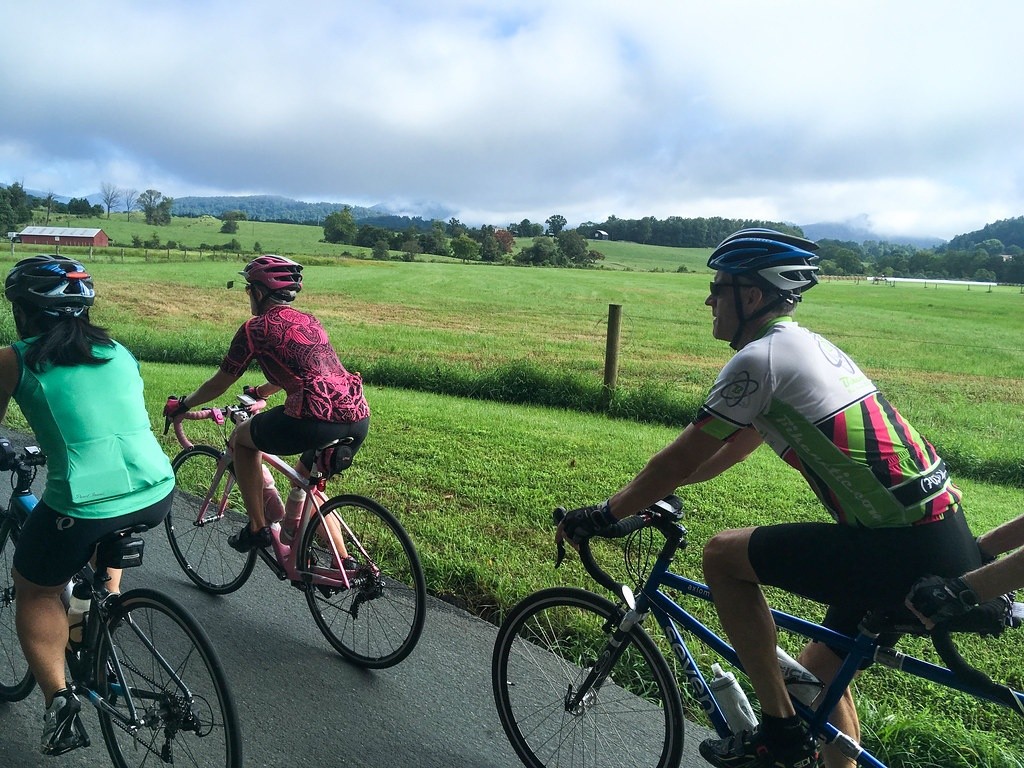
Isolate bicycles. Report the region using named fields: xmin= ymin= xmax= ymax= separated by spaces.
xmin=162 ymin=385 xmax=429 ymax=670
xmin=491 ymin=493 xmax=1024 ymax=768
xmin=0 ymin=436 xmax=243 ymax=768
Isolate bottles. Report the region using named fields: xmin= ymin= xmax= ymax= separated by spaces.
xmin=777 ymin=646 xmax=825 ymax=705
xmin=67 ymin=579 xmax=91 ymax=642
xmin=280 ymin=485 xmax=307 ymax=545
xmin=709 ymin=662 xmax=759 ymax=735
xmin=261 ymin=464 xmax=285 ymax=522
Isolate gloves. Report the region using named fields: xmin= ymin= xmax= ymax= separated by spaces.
xmin=243 ymin=385 xmax=269 ymax=402
xmin=163 ymin=396 xmax=190 ymax=417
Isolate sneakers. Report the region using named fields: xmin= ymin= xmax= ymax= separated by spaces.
xmin=41 ymin=688 xmax=81 ymax=755
xmin=330 ymin=555 xmax=357 ymax=571
xmin=699 ymin=724 xmax=817 ymax=768
xmin=227 ymin=522 xmax=273 ymax=552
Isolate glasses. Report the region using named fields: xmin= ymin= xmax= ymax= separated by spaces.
xmin=710 ymin=281 xmax=766 ymax=298
xmin=245 ymin=286 xmax=264 ymax=296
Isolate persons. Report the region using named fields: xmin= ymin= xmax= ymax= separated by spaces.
xmin=161 ymin=253 xmax=373 ymax=571
xmin=554 ymin=226 xmax=982 ymax=768
xmin=0 ymin=253 xmax=175 ymax=755
xmin=907 ymin=513 xmax=1024 ymax=630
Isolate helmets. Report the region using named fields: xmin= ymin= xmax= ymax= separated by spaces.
xmin=238 ymin=255 xmax=303 ymax=292
xmin=5 ymin=254 xmax=95 ymax=317
xmin=706 ymin=227 xmax=820 ymax=305
